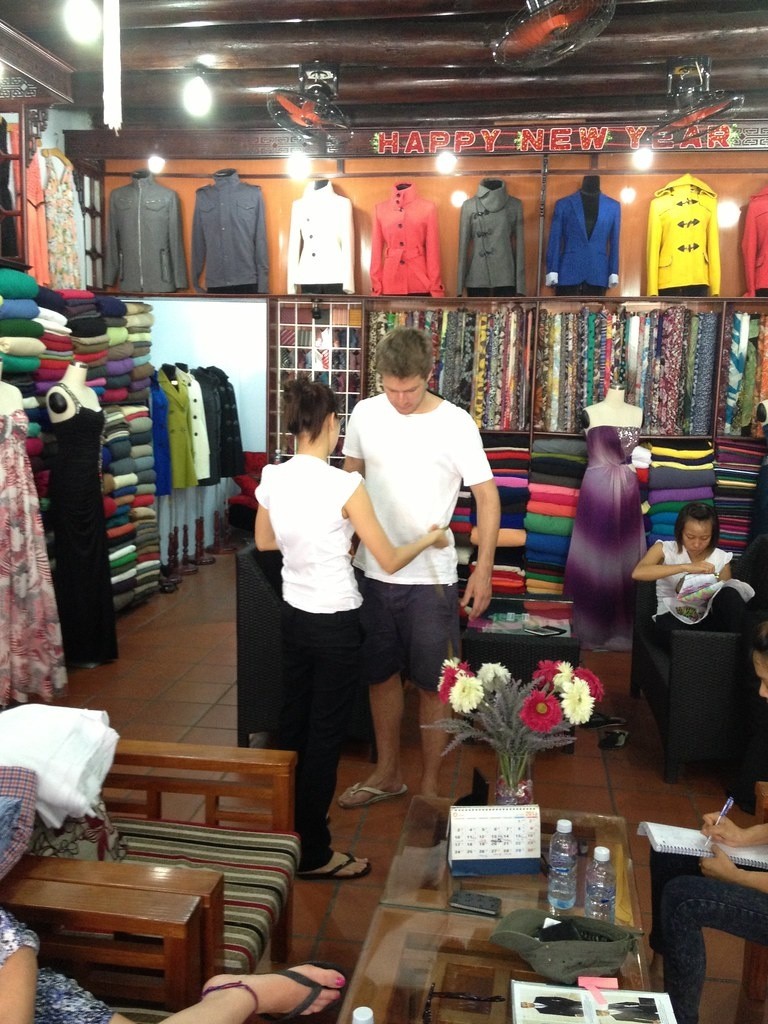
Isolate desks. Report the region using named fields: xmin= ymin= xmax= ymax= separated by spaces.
xmin=454 ymin=597 xmax=582 ymax=755
xmin=378 ymin=795 xmax=644 ymax=935
xmin=337 ymin=903 xmax=652 ymax=1024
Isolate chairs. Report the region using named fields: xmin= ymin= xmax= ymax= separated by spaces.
xmin=0 ymin=702 xmax=300 ymax=1024
xmin=234 ymin=542 xmax=408 ymax=764
xmin=628 ymin=534 xmax=768 ymax=783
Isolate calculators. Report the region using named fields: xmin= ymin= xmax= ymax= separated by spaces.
xmin=525 ymin=626 xmax=566 ymax=636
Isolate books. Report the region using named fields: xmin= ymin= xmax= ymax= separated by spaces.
xmin=637 ymin=821 xmax=768 ymax=869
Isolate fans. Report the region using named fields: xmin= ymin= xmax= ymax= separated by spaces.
xmin=490 ymin=0 xmax=617 ymax=72
xmin=645 ymin=56 xmax=744 ymax=145
xmin=263 ymin=61 xmax=354 ymax=149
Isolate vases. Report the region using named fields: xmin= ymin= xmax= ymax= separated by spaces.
xmin=493 ymin=774 xmax=534 ymax=806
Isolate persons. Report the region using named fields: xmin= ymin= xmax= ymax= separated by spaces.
xmin=40 ymin=358 xmax=120 ymax=668
xmin=0 ymin=905 xmax=346 ymax=1024
xmin=546 ymin=173 xmax=621 ymax=297
xmin=595 ymin=1001 xmax=662 ymax=1024
xmin=106 ymin=169 xmax=189 ymax=294
xmin=521 ymin=995 xmax=583 ymax=1018
xmin=566 ymin=382 xmax=648 ymax=652
xmin=190 ymin=364 xmax=246 ymax=554
xmin=370 ymin=182 xmax=446 ymax=298
xmin=148 ymin=360 xmax=212 ymax=571
xmin=648 ymin=624 xmax=768 ymax=1024
xmin=741 ymin=186 xmax=768 ymax=296
xmin=0 ymin=359 xmax=68 ymax=715
xmin=454 ymin=178 xmax=527 ymax=298
xmin=631 ymin=502 xmax=733 ymax=650
xmin=337 ymin=327 xmax=500 ymax=813
xmin=286 ymin=179 xmax=355 ymax=296
xmin=254 ymin=380 xmax=449 ymax=879
xmin=189 ymin=168 xmax=269 ymax=294
xmin=647 ymin=172 xmax=722 ymax=295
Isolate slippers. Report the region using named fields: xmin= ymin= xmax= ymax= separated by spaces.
xmin=203 ymin=961 xmax=346 ymax=1022
xmin=579 ymin=711 xmax=627 ymax=730
xmin=338 ymin=781 xmax=409 ymax=808
xmin=296 ymin=851 xmax=371 ymax=877
xmin=597 ymin=729 xmax=630 ymax=748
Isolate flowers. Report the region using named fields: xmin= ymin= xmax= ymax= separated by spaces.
xmin=420 ymin=656 xmax=606 ymax=787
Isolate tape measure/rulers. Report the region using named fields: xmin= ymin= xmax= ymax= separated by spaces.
xmin=426 ymin=525 xmax=457 ymax=660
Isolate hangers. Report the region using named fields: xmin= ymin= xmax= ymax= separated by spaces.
xmin=40 ymin=132 xmax=75 ymax=172
xmin=6 ymin=123 xmax=43 ymax=147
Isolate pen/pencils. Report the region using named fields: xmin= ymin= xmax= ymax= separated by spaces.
xmin=704 ymin=796 xmax=735 ymax=845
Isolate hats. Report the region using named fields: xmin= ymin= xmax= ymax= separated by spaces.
xmin=487 ymin=907 xmax=636 ymax=983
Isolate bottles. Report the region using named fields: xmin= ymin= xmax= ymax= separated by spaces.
xmin=584 ymin=846 xmax=615 ymax=924
xmin=547 ymin=819 xmax=577 ymax=910
xmin=351 ymin=1006 xmax=374 ymax=1024
